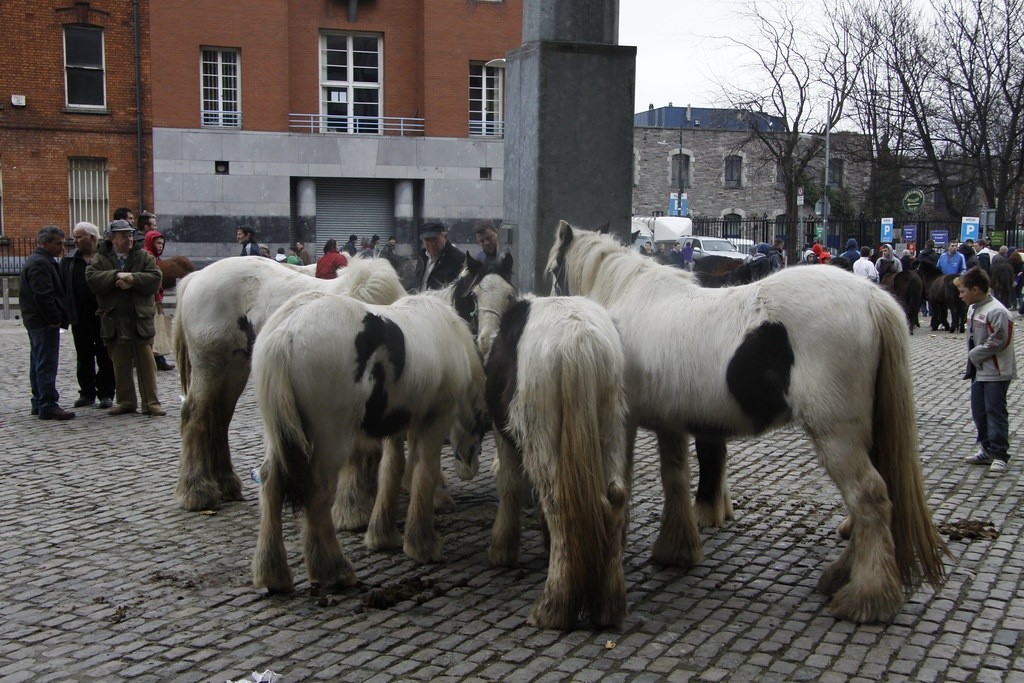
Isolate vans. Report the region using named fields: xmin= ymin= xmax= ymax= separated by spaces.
xmin=632 ymin=221 xmax=657 ymax=255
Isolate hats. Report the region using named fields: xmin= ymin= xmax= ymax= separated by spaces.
xmin=372 ymin=234 xmax=379 ymax=241
xmin=419 ymin=221 xmax=445 ymax=239
xmin=107 ymin=219 xmax=135 ymax=233
xmin=999 ymin=246 xmax=1009 ymax=252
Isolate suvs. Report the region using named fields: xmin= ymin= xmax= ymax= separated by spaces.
xmin=675 ymin=234 xmax=746 ymax=261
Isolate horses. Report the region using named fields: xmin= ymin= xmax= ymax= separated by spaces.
xmin=990 ymin=261 xmax=1014 ymax=310
xmin=156 ymin=254 xmax=409 ymax=511
xmin=254 ymin=280 xmax=486 ymax=606
xmin=879 ymin=259 xmax=922 ymax=336
xmin=693 ymin=256 xmax=773 ymax=289
xmin=456 ymin=254 xmax=631 ymax=632
xmin=911 ymin=259 xmax=969 ymax=333
xmin=546 ymin=216 xmax=954 ymax=627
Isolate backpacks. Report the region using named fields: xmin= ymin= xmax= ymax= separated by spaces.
xmin=340 ymin=242 xmax=350 ymax=253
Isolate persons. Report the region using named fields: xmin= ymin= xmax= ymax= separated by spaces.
xmin=341 ymin=235 xmax=396 ymax=260
xmin=315 ymin=239 xmax=348 ymax=280
xmin=743 ymin=238 xmax=784 ymax=283
xmin=237 ymin=224 xmax=261 ymax=256
xmin=86 ymin=220 xmax=167 ymax=416
xmin=19 ymin=226 xmax=75 ymax=419
xmin=103 ymin=208 xmax=135 ymax=241
xmin=955 ymin=266 xmax=1018 ymax=471
xmin=474 ymin=220 xmax=498 ymax=265
xmin=143 ymin=232 xmax=175 ymax=372
xmin=61 ymin=222 xmax=116 ymax=408
xmin=798 ymin=238 xmax=1024 ymax=330
xmin=134 ymin=212 xmax=157 ymax=249
xmin=644 ymin=241 xmax=693 ymax=270
xmin=258 ymin=241 xmax=312 ymax=266
xmin=414 ymin=220 xmax=467 ymax=293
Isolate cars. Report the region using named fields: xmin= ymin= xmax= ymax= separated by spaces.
xmin=724 ymin=237 xmax=760 ymax=252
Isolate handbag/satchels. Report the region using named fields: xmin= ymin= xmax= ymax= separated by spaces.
xmin=152 ymin=304 xmax=174 ymax=356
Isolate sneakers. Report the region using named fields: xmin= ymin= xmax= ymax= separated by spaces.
xmin=966 ymin=449 xmax=994 ymax=464
xmin=991 ymin=458 xmax=1008 ymax=471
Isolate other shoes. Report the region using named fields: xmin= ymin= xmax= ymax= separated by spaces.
xmin=158 ymin=363 xmax=176 ymax=371
xmin=99 ymin=397 xmax=113 ymax=408
xmin=108 ymin=405 xmax=136 ymax=415
xmin=74 ymin=395 xmax=96 ymax=406
xmin=31 ymin=404 xmax=39 ymax=414
xmin=39 ymin=407 xmax=75 ymax=420
xmin=145 ymin=409 xmax=167 ymax=416
